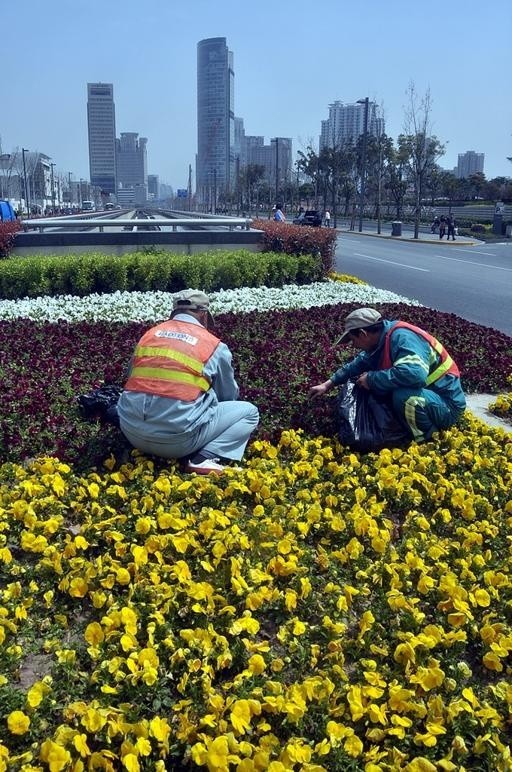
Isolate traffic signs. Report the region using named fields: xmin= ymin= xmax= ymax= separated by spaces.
xmin=177 ymin=189 xmax=188 ymax=198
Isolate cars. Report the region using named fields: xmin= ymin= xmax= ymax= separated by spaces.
xmin=116 ymin=205 xmax=121 ymax=209
xmin=96 ymin=207 xmax=105 ymax=212
xmin=209 ymin=207 xmax=229 ymax=213
xmin=292 ymin=209 xmax=323 ymax=227
xmin=42 ymin=205 xmax=80 ymax=215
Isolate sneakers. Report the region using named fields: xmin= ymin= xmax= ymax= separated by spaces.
xmin=183 ymin=457 xmax=245 ymax=477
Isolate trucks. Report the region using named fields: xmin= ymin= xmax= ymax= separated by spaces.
xmin=0 ymin=199 xmax=17 ymax=222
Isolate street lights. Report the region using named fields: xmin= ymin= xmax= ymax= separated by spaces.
xmin=68 ymin=170 xmax=73 ymax=186
xmin=22 ymin=147 xmax=29 ymax=218
xmin=50 ymin=162 xmax=56 ymax=206
xmin=271 ymin=136 xmax=280 ymax=206
xmin=79 ymin=177 xmax=83 ymax=201
xmin=356 ymin=96 xmax=373 ymax=233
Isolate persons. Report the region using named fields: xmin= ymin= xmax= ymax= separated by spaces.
xmin=306 ymin=308 xmax=466 ymax=449
xmin=273 ymin=202 xmax=330 ymax=227
xmin=430 ymin=213 xmax=456 ymax=240
xmin=116 ymin=291 xmax=260 ymax=476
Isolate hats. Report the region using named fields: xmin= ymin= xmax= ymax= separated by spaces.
xmin=170 ymin=289 xmax=216 ymax=331
xmin=335 ymin=307 xmax=384 ymax=346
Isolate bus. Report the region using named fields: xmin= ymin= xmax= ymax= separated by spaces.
xmin=81 ymin=200 xmax=96 ymax=213
xmin=105 ymin=202 xmax=114 ymax=211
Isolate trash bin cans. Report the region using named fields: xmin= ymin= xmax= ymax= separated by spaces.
xmin=242 ymin=211 xmax=247 ymax=218
xmin=391 ymin=221 xmax=402 ymax=236
